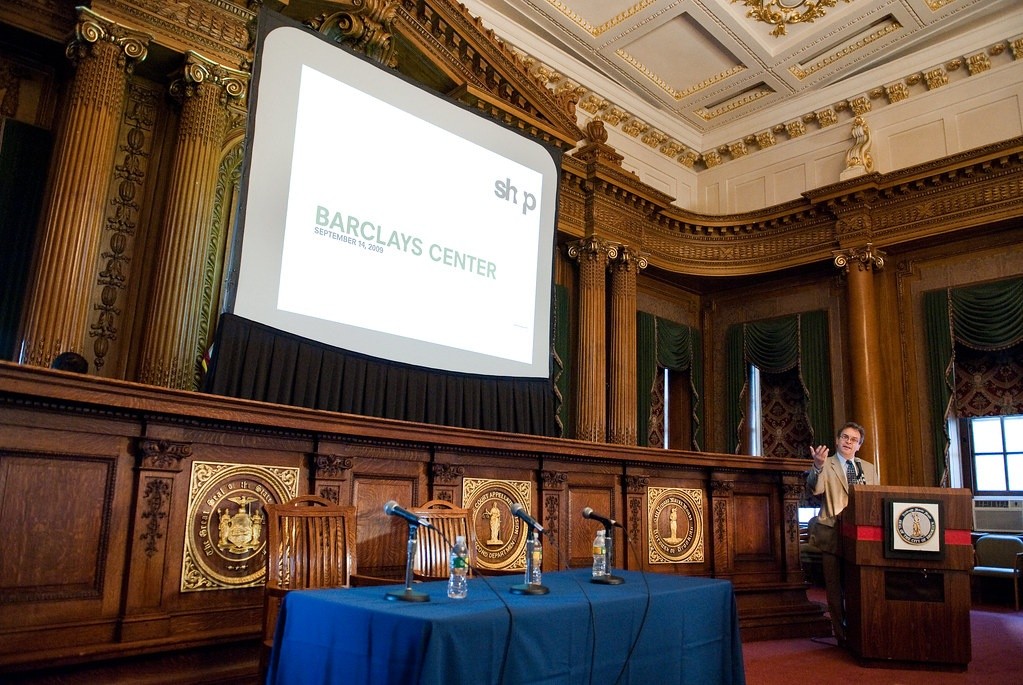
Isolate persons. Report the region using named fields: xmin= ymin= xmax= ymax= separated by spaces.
xmin=805 ymin=422 xmax=878 ymax=651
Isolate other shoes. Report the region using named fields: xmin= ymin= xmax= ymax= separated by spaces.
xmin=837 ymin=639 xmax=847 ymax=648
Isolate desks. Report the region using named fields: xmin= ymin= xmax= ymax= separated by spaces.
xmin=266 ymin=570 xmax=744 ymax=685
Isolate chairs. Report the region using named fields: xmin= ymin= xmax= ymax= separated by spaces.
xmin=971 ymin=534 xmax=1023 ymax=613
xmin=258 ymin=494 xmax=422 ymax=684
xmin=404 ymin=499 xmax=526 ymax=587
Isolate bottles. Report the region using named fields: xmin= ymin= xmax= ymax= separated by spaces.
xmin=524 ymin=533 xmax=542 ymax=585
xmin=447 ymin=536 xmax=468 ymax=599
xmin=592 ymin=530 xmax=606 ymax=576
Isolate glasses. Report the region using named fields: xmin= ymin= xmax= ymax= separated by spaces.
xmin=840 ymin=433 xmax=860 ymax=445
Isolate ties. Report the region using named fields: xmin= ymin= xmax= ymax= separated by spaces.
xmin=845 ymin=460 xmax=858 ymax=485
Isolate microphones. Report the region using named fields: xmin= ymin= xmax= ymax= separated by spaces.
xmin=383 ymin=500 xmax=434 ymax=529
xmin=582 ymin=507 xmax=622 ymax=527
xmin=855 ymin=457 xmax=866 ymax=485
xmin=511 ymin=503 xmax=548 ymax=535
